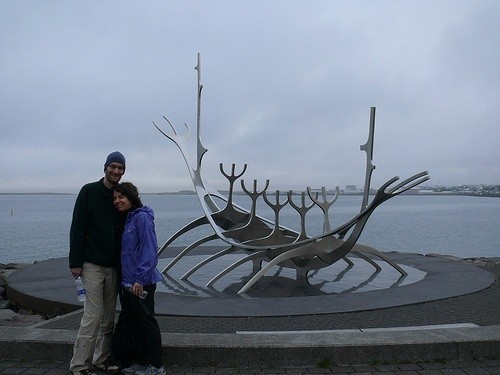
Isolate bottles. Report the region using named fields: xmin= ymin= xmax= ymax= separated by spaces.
xmin=76 ymin=276 xmax=86 ymax=302
xmin=121 ymin=282 xmax=148 ymax=299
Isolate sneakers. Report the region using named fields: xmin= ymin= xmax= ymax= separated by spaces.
xmin=122 ymin=364 xmax=144 ymax=375
xmin=135 ymin=364 xmax=167 ymax=375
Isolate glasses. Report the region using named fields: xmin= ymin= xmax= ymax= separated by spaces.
xmin=108 ymin=165 xmax=124 ymax=170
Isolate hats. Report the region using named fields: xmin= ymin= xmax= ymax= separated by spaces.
xmin=104 ymin=151 xmax=125 ymax=174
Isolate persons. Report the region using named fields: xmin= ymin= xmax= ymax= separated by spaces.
xmin=69 ymin=151 xmax=126 ymax=375
xmin=112 ymin=182 xmax=167 ymax=375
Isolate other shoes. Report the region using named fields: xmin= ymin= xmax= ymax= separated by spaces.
xmin=70 ymin=369 xmax=99 ymax=375
xmin=96 ymin=359 xmax=122 ymax=375
